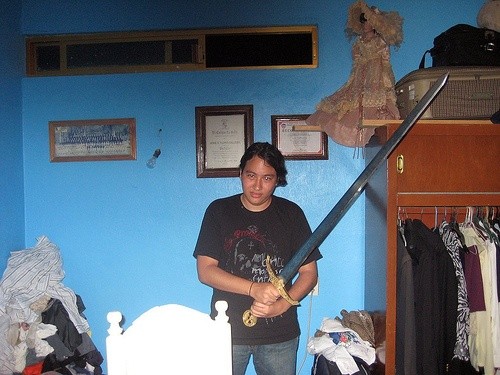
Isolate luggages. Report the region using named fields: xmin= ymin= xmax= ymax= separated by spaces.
xmin=395 ymin=66 xmax=500 ymax=120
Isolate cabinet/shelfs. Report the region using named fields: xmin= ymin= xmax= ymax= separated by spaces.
xmin=364 ymin=116 xmax=499 ymax=375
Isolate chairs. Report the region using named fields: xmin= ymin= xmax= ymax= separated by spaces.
xmin=105 ymin=299 xmax=235 ymax=374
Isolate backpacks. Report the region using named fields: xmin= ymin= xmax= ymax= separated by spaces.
xmin=428 ymin=24 xmax=500 ymax=68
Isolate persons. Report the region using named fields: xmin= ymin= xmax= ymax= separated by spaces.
xmin=193 ymin=142 xmax=323 ymax=375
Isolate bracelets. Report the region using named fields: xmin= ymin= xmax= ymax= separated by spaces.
xmin=248 ymin=281 xmax=255 ymax=297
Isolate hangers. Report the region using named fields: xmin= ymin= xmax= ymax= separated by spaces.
xmin=398 ymin=202 xmax=500 ymax=246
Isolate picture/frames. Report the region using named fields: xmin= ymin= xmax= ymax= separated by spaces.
xmin=48 ymin=119 xmax=139 ymax=162
